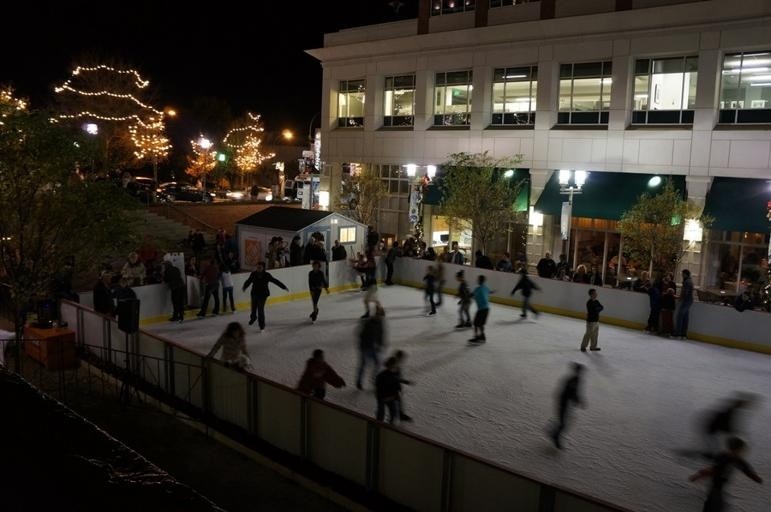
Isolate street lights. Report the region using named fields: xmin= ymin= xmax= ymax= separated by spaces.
xmin=554 ymin=168 xmax=594 ymax=270
xmin=401 ymin=162 xmax=439 ymax=257
xmin=270 ymin=128 xmax=294 ymax=163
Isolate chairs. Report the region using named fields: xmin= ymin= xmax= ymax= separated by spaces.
xmin=696 ymin=289 xmax=710 ymax=301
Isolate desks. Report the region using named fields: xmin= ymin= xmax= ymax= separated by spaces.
xmin=707 ymin=288 xmax=739 ymax=305
xmin=22 ymin=322 xmax=77 ymax=369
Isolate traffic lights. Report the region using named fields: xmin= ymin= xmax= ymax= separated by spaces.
xmin=217 ymin=152 xmax=227 ymax=174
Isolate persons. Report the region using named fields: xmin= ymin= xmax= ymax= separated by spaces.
xmin=688 ymin=392 xmax=770 ymax=510
xmin=308 ymin=260 xmax=330 ymax=323
xmin=121 ymin=251 xmax=145 ymax=289
xmin=384 ymin=241 xmax=401 ymax=285
xmin=547 ymin=364 xmax=587 ymax=453
xmin=355 ymin=308 xmax=416 ymax=422
xmin=162 ymin=251 xmax=237 ymax=322
xmin=266 ymin=232 xmax=327 ymax=269
xmin=350 ymin=249 xmax=381 ymax=319
xmin=364 ymin=226 xmax=379 ymax=254
xmin=296 ymin=349 xmax=346 ymax=400
xmin=580 ymin=289 xmax=604 ymax=352
xmin=422 ymin=258 xmax=495 ymax=344
xmin=510 ymin=268 xmax=541 ymax=318
xmin=205 ymin=321 xmax=252 ymax=371
xmin=719 ymin=250 xmax=769 ymax=312
xmin=645 ymin=269 xmax=694 ymax=342
xmin=402 ymin=235 xmax=514 ymax=274
xmin=250 ymin=185 xmax=258 ymax=202
xmin=92 ymin=270 xmax=137 ymax=319
xmin=537 ymin=253 xmax=651 ymax=294
xmin=331 ymin=240 xmax=347 ymax=262
xmin=242 ymin=260 xmax=288 ymax=330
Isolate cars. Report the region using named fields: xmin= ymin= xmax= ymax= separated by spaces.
xmin=70 ymin=165 xmax=319 ymax=208
